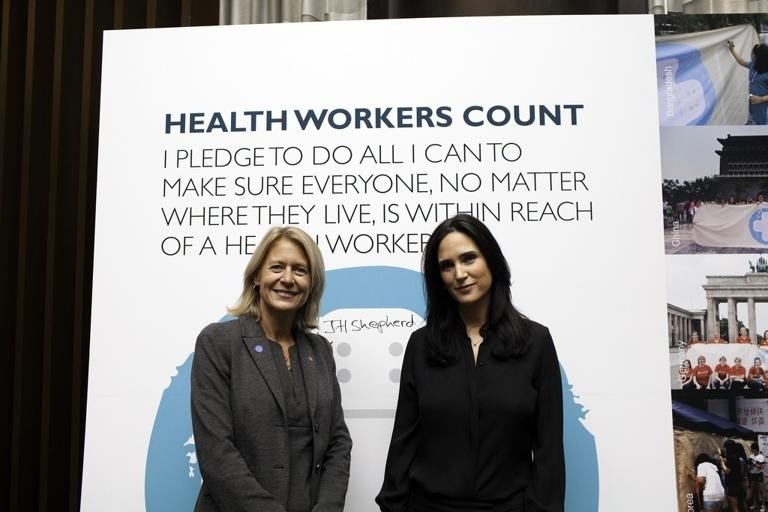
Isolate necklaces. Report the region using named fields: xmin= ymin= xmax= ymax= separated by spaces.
xmin=470 ymin=338 xmax=481 ymax=346
xmin=282 ymin=344 xmax=292 ymax=362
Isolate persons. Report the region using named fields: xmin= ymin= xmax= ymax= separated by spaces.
xmin=190 ymin=226 xmax=352 ymax=512
xmin=374 ymin=215 xmax=564 ymax=512
xmin=726 ymin=39 xmax=768 ymax=125
xmin=686 ymin=440 xmax=766 ymax=512
xmin=679 ymin=326 xmax=767 ymax=387
xmin=665 ymin=193 xmax=767 ymax=226
